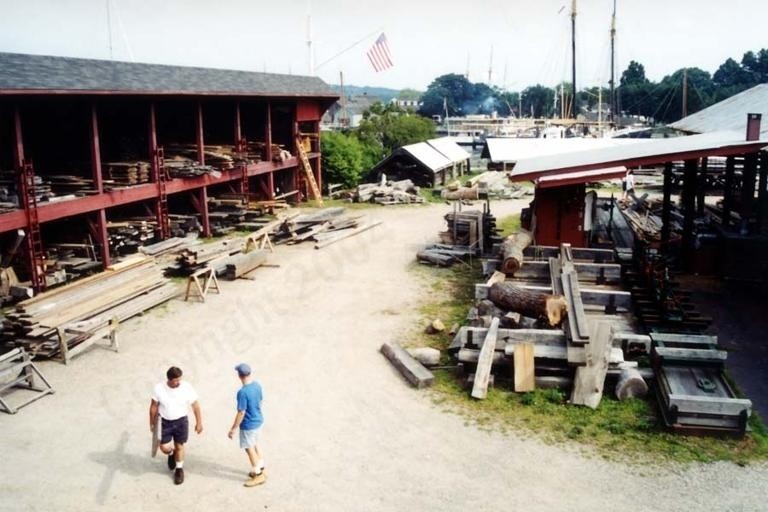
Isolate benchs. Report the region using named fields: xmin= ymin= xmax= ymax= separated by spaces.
xmin=246 ymin=231 xmax=275 ymax=253
xmin=183 ymin=266 xmax=220 ymax=302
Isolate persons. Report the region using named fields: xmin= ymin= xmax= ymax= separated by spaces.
xmin=620 ymin=168 xmax=633 ymax=198
xmin=625 ymin=170 xmax=636 ymax=199
xmin=583 ymin=124 xmax=589 ymax=137
xmin=227 ymin=362 xmax=268 ymax=488
xmin=149 ymin=366 xmax=204 ymax=485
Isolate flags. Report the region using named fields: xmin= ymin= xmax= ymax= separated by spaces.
xmin=365 ymin=32 xmax=396 ymax=73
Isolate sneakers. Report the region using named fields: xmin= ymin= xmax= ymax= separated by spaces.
xmin=175 ymin=469 xmax=184 ymax=484
xmin=245 ymin=468 xmax=266 ymax=487
xmin=168 ymin=454 xmax=175 ymax=470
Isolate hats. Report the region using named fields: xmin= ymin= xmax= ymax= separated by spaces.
xmin=235 ymin=364 xmax=251 ymax=375
